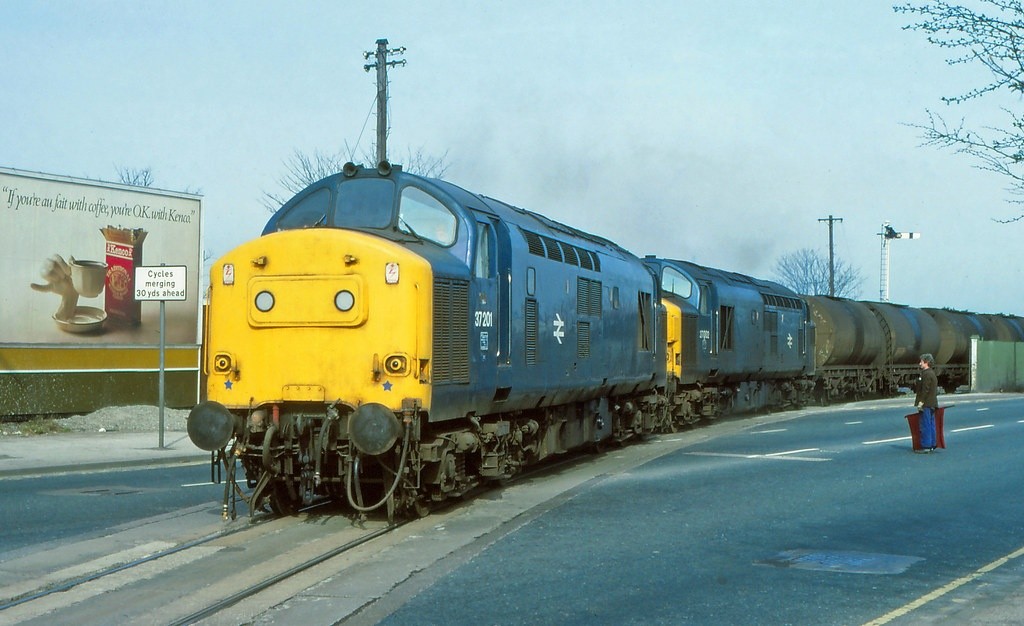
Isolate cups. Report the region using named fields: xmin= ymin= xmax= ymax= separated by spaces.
xmin=71 ymin=260 xmax=107 ymax=298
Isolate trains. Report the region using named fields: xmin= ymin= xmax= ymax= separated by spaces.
xmin=187 ymin=161 xmax=1024 ymax=528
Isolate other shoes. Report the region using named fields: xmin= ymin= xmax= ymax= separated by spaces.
xmin=915 ymin=449 xmax=935 ymax=454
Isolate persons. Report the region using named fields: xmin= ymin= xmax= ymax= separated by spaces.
xmin=914 ymin=354 xmax=939 ymax=454
